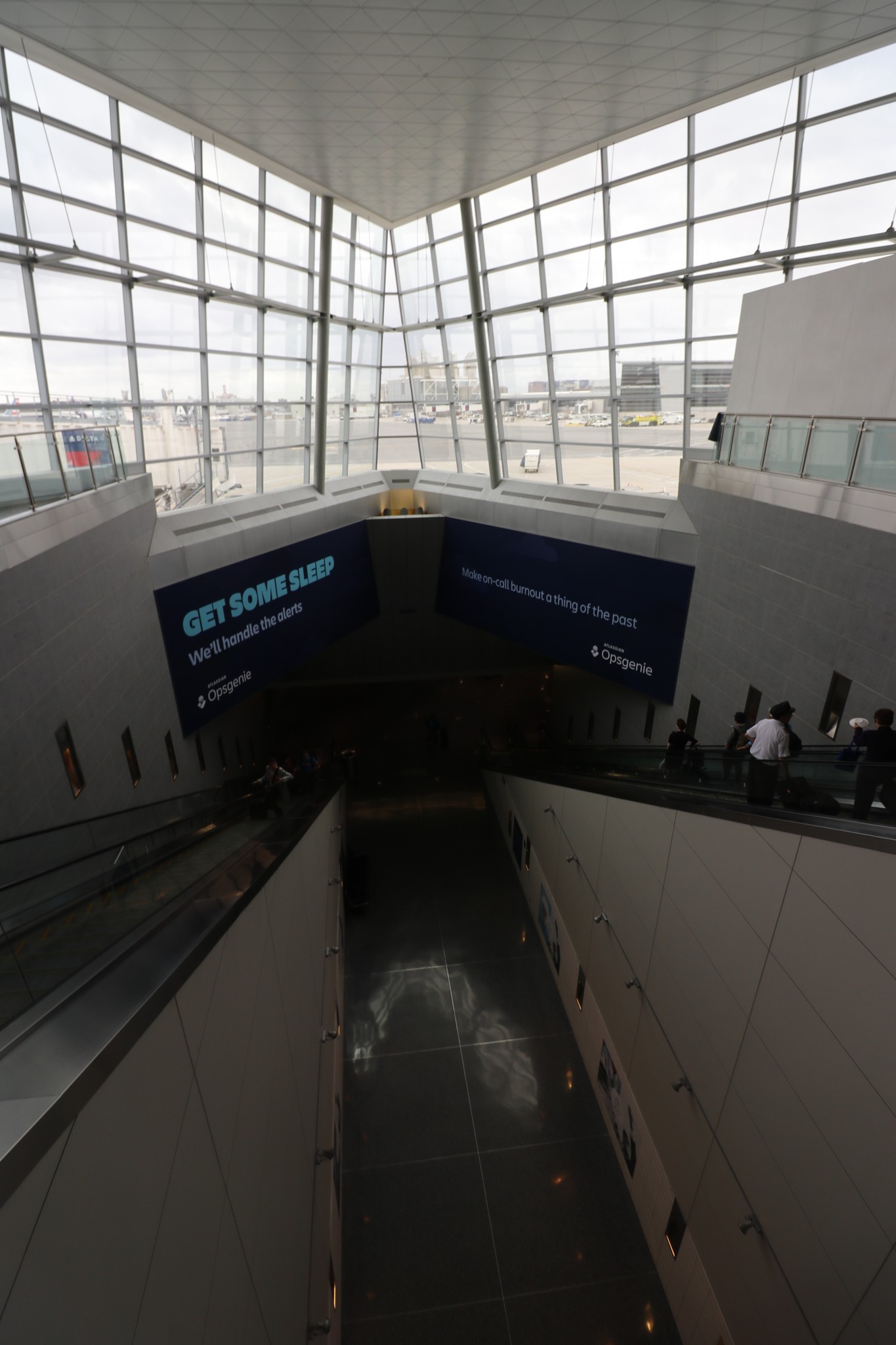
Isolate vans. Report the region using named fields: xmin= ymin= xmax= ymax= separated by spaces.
xmin=664 ymin=415 xmax=683 ymax=425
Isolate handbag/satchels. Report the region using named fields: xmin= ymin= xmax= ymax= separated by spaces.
xmin=659 ymin=758 xmax=666 ymax=770
xmin=832 ymin=735 xmax=861 ymax=773
xmin=722 ymin=728 xmax=740 ymax=759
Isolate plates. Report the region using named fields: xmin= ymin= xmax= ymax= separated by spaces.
xmin=849 ymin=717 xmax=869 ymax=728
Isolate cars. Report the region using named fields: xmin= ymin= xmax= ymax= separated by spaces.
xmin=568 ymin=413 xmax=609 ymax=427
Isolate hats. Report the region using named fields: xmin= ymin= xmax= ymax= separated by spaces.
xmin=775 ymin=701 xmax=796 ymax=716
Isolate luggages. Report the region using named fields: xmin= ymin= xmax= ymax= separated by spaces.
xmin=776 ymin=774 xmax=817 ymax=816
xmin=797 ymin=792 xmax=843 ymax=817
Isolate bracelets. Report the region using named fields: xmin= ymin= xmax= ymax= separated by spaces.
xmin=745 ymin=744 xmax=747 ymax=747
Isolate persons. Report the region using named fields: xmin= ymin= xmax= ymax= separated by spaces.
xmin=720 ymin=701 xmax=796 ymax=807
xmin=664 ymin=718 xmax=700 ymax=777
xmin=851 ymin=709 xmax=896 ymax=820
xmin=252 ymin=750 xmax=318 ymax=819
xmin=425 ymin=713 xmax=441 ymax=746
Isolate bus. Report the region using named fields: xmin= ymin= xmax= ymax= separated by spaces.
xmin=619 ymin=415 xmax=663 ymax=428
